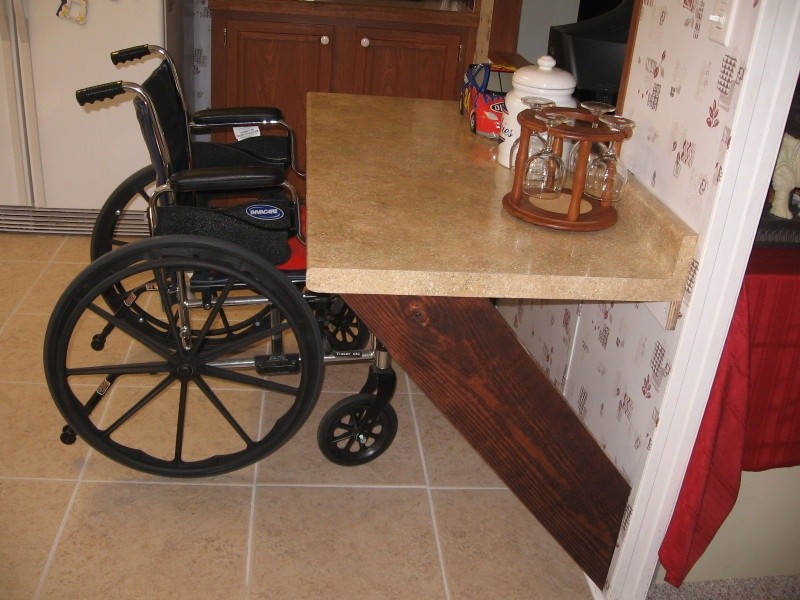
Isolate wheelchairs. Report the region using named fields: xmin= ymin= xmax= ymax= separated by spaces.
xmin=41 ymin=42 xmax=400 ymax=480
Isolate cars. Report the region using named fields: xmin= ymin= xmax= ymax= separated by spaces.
xmin=458 ymin=62 xmax=519 ymax=141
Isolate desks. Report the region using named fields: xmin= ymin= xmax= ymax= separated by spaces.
xmin=304 ymin=91 xmax=701 ymax=591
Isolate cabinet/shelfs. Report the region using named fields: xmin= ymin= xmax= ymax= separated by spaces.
xmin=208 ymin=0 xmax=481 ymax=208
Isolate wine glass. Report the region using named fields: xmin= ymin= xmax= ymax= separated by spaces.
xmin=584 ymin=117 xmax=635 ymax=201
xmin=524 ymin=113 xmax=571 ymax=200
xmin=566 ymin=102 xmax=616 ymax=178
xmin=508 ymin=96 xmax=555 ymax=175
